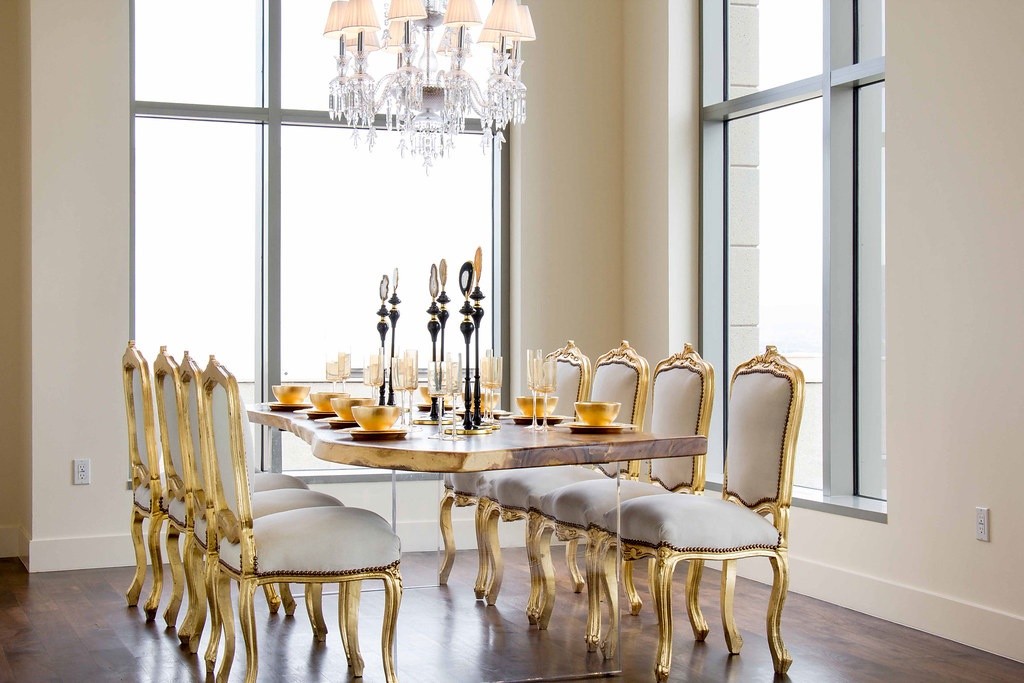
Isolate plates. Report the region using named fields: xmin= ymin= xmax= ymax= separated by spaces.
xmin=554 ymin=421 xmax=636 ymax=433
xmin=338 ymin=428 xmax=408 ymax=441
xmin=456 ymin=411 xmax=510 ymax=418
xmin=304 ymin=411 xmax=337 ymax=418
xmin=416 ymin=404 xmax=458 ymax=412
xmin=314 ymin=416 xmax=357 ymax=426
xmin=512 ymin=416 xmax=563 ymax=426
xmin=263 ymin=402 xmax=312 ymax=412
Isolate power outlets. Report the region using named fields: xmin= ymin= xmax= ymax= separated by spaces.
xmin=975 ymin=506 xmax=990 ymax=543
xmin=73 ymin=458 xmax=91 ymax=485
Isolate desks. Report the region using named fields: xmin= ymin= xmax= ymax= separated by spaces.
xmin=247 ymin=399 xmax=707 ymax=683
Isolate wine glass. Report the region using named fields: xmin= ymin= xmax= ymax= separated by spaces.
xmin=533 ymin=358 xmax=558 ymax=432
xmin=525 ymin=349 xmax=543 ymax=429
xmin=326 ymin=345 xmax=503 ymax=442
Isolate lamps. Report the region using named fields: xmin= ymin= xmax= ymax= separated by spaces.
xmin=323 ymin=0 xmax=537 ymax=176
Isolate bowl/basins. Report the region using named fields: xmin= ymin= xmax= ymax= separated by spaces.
xmin=309 ymin=393 xmax=350 ymax=411
xmin=272 ymin=384 xmax=310 ymax=403
xmin=330 ymin=397 xmax=375 ymax=420
xmin=574 ymin=401 xmax=621 ymax=425
xmin=419 ymin=386 xmax=452 ymax=404
xmin=351 ymin=406 xmax=400 ymax=429
xmin=514 ymin=397 xmax=559 ymax=415
xmin=461 ymin=392 xmax=500 ymax=411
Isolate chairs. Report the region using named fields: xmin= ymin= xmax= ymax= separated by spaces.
xmin=200 ymin=354 xmax=403 ymax=683
xmin=534 ymin=342 xmax=717 ymax=652
xmin=436 ymin=343 xmax=590 ymax=597
xmin=481 ymin=343 xmax=652 ymax=623
xmin=121 ymin=338 xmax=281 ymax=619
xmin=599 ymin=343 xmax=806 ymax=679
xmin=152 ymin=347 xmax=319 ymax=639
xmin=179 ymin=351 xmax=354 ymax=667
